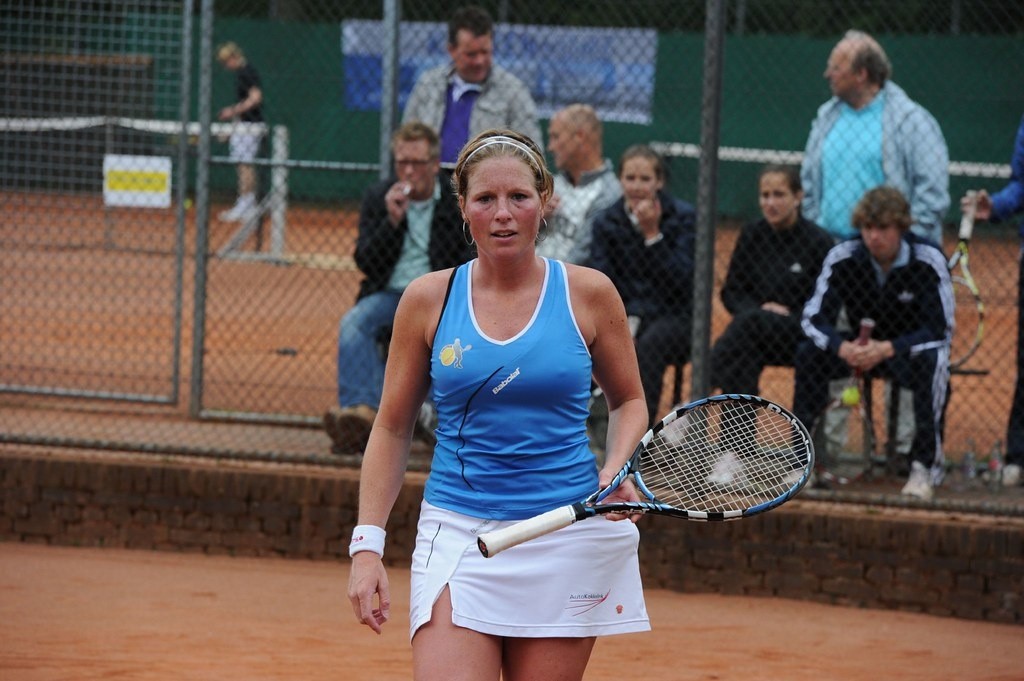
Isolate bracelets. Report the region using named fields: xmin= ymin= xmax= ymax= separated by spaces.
xmin=347 ymin=526 xmax=386 ymax=559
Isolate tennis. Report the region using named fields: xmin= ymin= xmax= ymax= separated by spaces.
xmin=843 ymin=387 xmax=859 ymax=406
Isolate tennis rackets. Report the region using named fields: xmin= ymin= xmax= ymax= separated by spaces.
xmin=809 ymin=319 xmax=876 ymax=487
xmin=948 ymin=190 xmax=984 ymax=369
xmin=477 ymin=394 xmax=814 ymax=558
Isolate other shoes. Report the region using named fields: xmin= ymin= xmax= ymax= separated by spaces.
xmin=659 ymin=406 xmax=690 ymax=449
xmin=704 ymin=454 xmax=749 ymax=486
xmin=902 ymin=461 xmax=935 ymax=503
xmin=325 ymin=405 xmax=383 ymax=459
xmin=414 ymin=402 xmax=438 ymax=446
xmin=219 ymin=194 xmax=261 ymax=223
xmin=782 ymin=469 xmax=818 ymax=489
xmin=1001 ymin=464 xmax=1024 ymax=487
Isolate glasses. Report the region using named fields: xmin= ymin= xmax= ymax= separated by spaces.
xmin=393 ymin=156 xmax=433 ymax=169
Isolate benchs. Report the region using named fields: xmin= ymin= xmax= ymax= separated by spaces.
xmin=669 ymin=347 xmax=992 ymax=484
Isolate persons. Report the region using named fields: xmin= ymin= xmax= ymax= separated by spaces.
xmin=801 ymin=186 xmax=956 ymax=506
xmin=962 ymin=122 xmax=1024 ymax=488
xmin=322 ymin=119 xmax=478 ymax=447
xmin=590 ymin=145 xmax=703 ymax=447
xmin=530 ymin=104 xmax=620 ymax=268
xmin=219 ymin=41 xmax=266 ymax=220
xmin=402 ymin=9 xmax=547 ymax=175
xmin=344 ymin=129 xmax=650 ymax=681
xmin=703 ymin=162 xmax=834 ymax=452
xmin=798 ymin=31 xmax=949 ymax=482
